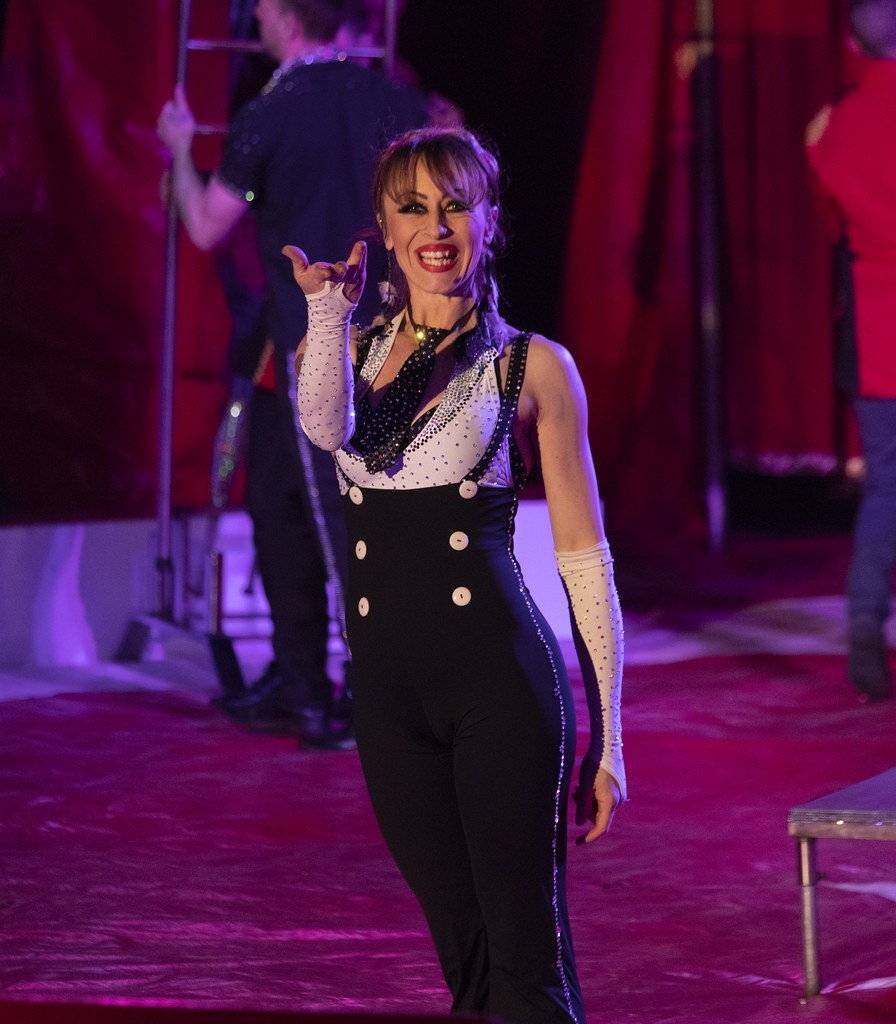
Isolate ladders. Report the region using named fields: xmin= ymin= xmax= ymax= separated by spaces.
xmin=155 ymin=0 xmax=397 ymax=570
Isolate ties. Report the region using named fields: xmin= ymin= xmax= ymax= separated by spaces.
xmin=359 ymin=323 xmax=448 ymax=474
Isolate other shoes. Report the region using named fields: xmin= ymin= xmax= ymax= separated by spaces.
xmin=847 ymin=625 xmax=891 ymax=699
xmin=322 ymin=662 xmax=357 ymax=750
xmin=213 ymin=679 xmax=333 ymax=738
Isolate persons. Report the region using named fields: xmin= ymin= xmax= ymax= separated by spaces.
xmin=277 ymin=126 xmax=634 ymax=1018
xmin=155 ymin=0 xmax=432 ymax=752
xmin=806 ymin=0 xmax=896 ymax=699
